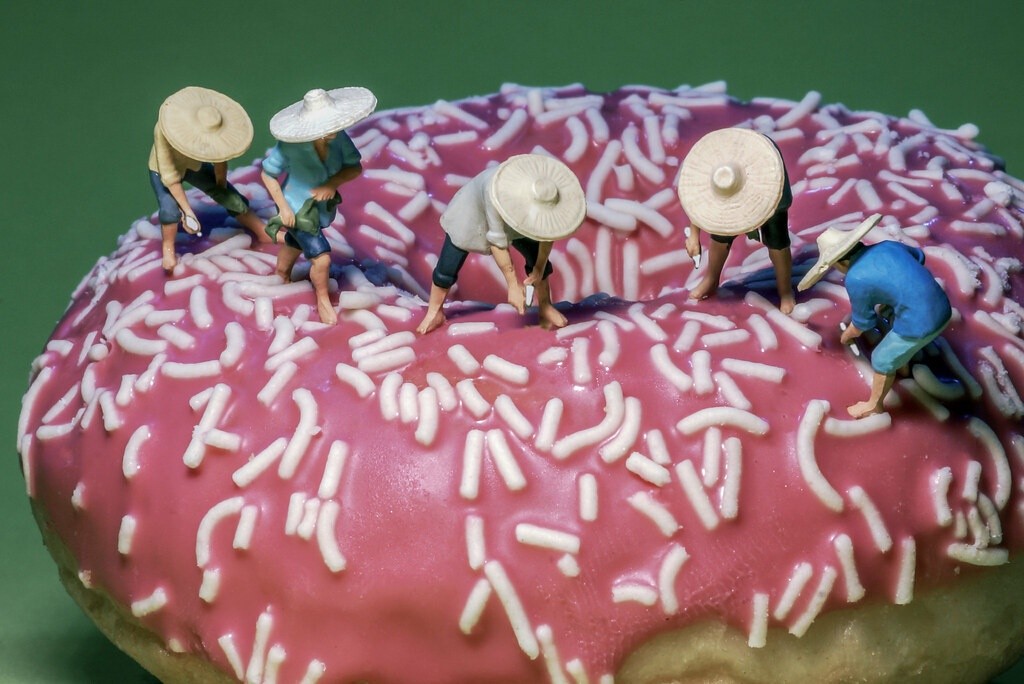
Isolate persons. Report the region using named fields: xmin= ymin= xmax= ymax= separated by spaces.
xmin=416 ymin=154 xmax=587 ymax=336
xmin=678 ymin=128 xmax=797 ymax=314
xmin=797 ymin=212 xmax=952 ymax=419
xmin=148 ymin=86 xmax=271 ymax=270
xmin=261 ymin=86 xmax=377 ymax=326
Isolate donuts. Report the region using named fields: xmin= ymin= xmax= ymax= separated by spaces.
xmin=12 ymin=81 xmax=1024 ymax=684
